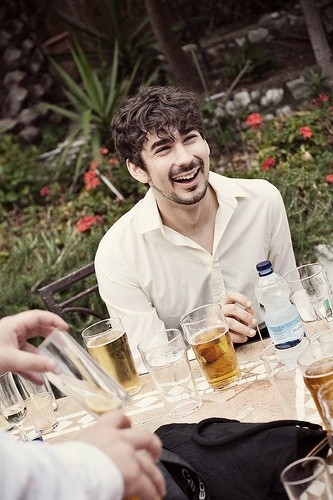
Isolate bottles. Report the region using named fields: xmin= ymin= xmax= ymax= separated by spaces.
xmin=255 ymin=261 xmax=308 ymax=360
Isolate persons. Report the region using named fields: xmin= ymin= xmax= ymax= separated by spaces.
xmin=0 ymin=308 xmax=166 ymax=500
xmin=94 ymin=85 xmax=303 ymax=379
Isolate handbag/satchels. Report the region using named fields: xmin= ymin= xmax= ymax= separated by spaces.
xmin=151 ymin=417 xmax=330 ymax=500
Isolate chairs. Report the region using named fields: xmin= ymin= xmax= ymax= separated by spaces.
xmin=38 ymin=262 xmax=108 ymax=379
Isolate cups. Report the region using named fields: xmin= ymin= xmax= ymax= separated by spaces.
xmin=283 ymin=263 xmax=333 ymax=341
xmin=34 ymin=326 xmax=131 ymax=419
xmin=136 ymin=328 xmax=202 ymax=418
xmin=180 ymin=302 xmax=241 ymax=390
xmin=81 ymin=317 xmax=142 ymax=396
xmin=280 ymin=456 xmax=333 ymax=500
xmin=15 ymin=369 xmax=58 ymax=412
xmin=260 ymin=330 xmax=333 ymax=445
xmin=24 ymin=393 xmax=58 ymax=435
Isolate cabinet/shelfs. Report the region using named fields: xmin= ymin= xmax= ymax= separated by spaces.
xmin=182 ymin=13 xmax=319 ymax=172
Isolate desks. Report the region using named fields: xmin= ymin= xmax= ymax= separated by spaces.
xmin=0 ymin=338 xmax=333 ymax=460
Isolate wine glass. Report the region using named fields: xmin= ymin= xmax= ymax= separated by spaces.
xmin=0 ymin=369 xmax=31 ymax=443
